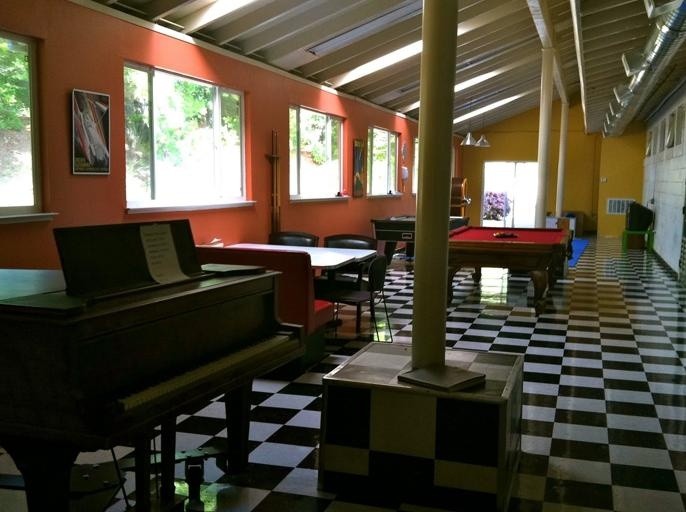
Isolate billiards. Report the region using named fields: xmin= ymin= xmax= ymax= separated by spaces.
xmin=493 ymin=232 xmax=517 ymax=238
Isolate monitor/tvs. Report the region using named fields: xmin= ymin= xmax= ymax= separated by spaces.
xmin=625 ymin=201 xmax=655 ymax=231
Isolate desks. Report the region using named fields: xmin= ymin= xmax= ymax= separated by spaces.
xmin=447 ymin=224 xmax=572 ymax=313
xmin=224 ymin=243 xmax=378 ymax=320
xmin=370 ymin=214 xmax=470 ymax=265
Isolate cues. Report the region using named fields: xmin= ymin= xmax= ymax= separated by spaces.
xmin=449 ymin=240 xmax=536 ymax=245
xmin=271 ymin=127 xmax=281 ymax=234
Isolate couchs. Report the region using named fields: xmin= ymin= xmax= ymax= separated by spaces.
xmin=196 ymin=246 xmax=334 ymax=338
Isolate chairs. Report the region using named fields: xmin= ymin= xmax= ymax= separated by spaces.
xmin=269 ymin=232 xmax=394 ymax=342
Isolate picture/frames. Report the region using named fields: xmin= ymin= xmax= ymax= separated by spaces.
xmin=353 ymin=139 xmax=364 ymax=198
xmin=72 ymin=88 xmax=111 ymax=176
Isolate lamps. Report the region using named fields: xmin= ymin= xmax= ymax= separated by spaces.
xmin=475 ymin=113 xmax=491 ymax=147
xmin=460 ymin=102 xmax=479 ymax=146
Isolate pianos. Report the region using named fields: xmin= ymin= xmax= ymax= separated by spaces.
xmin=1 ymin=218 xmax=306 ymax=511
